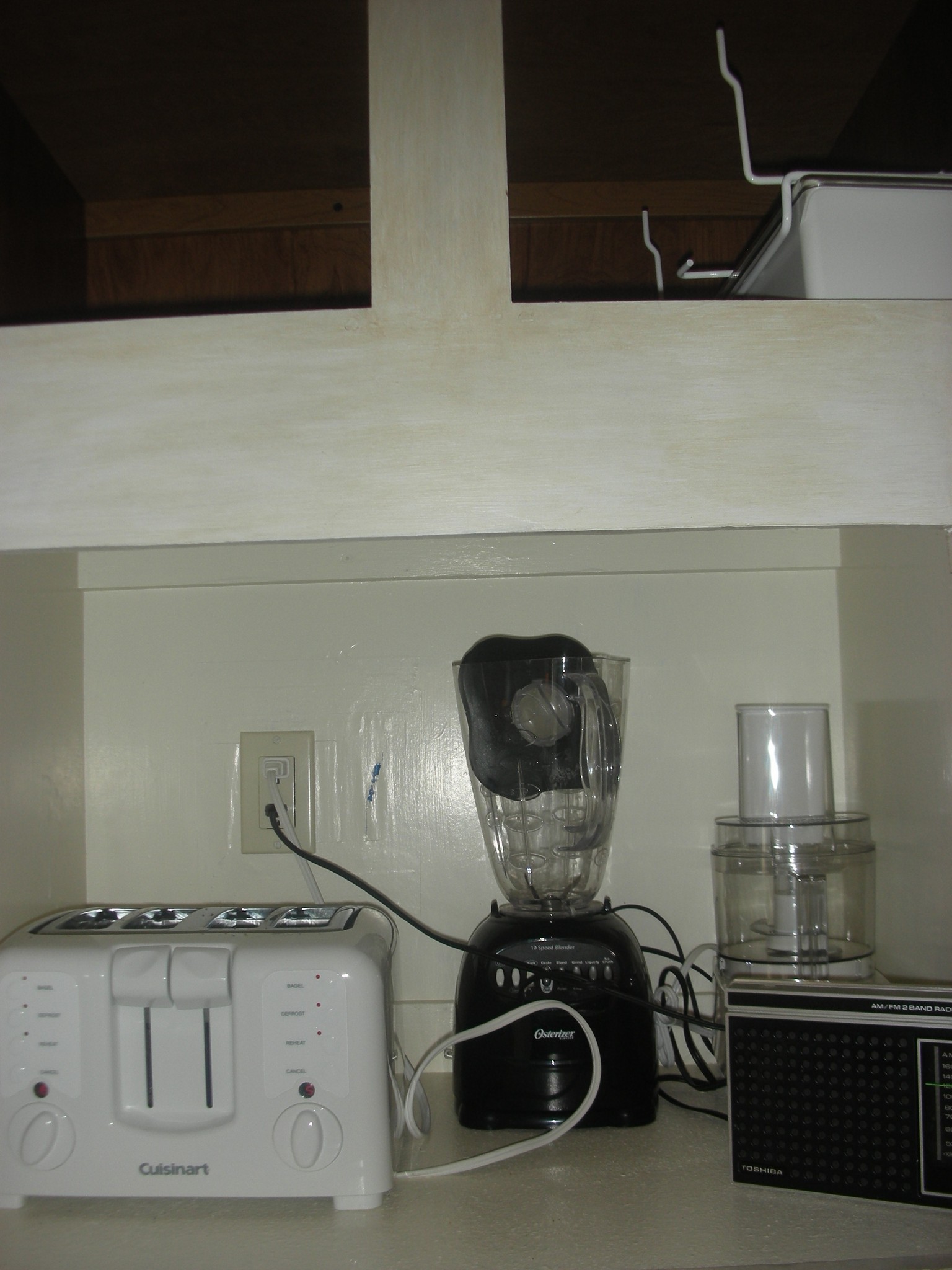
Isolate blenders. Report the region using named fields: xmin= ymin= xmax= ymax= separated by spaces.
xmin=452 ymin=633 xmax=657 ymax=1126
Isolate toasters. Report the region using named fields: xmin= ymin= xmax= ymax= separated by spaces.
xmin=1 ymin=905 xmax=394 ymax=1209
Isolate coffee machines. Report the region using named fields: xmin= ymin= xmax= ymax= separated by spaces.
xmin=708 ymin=702 xmax=893 ymax=1075
